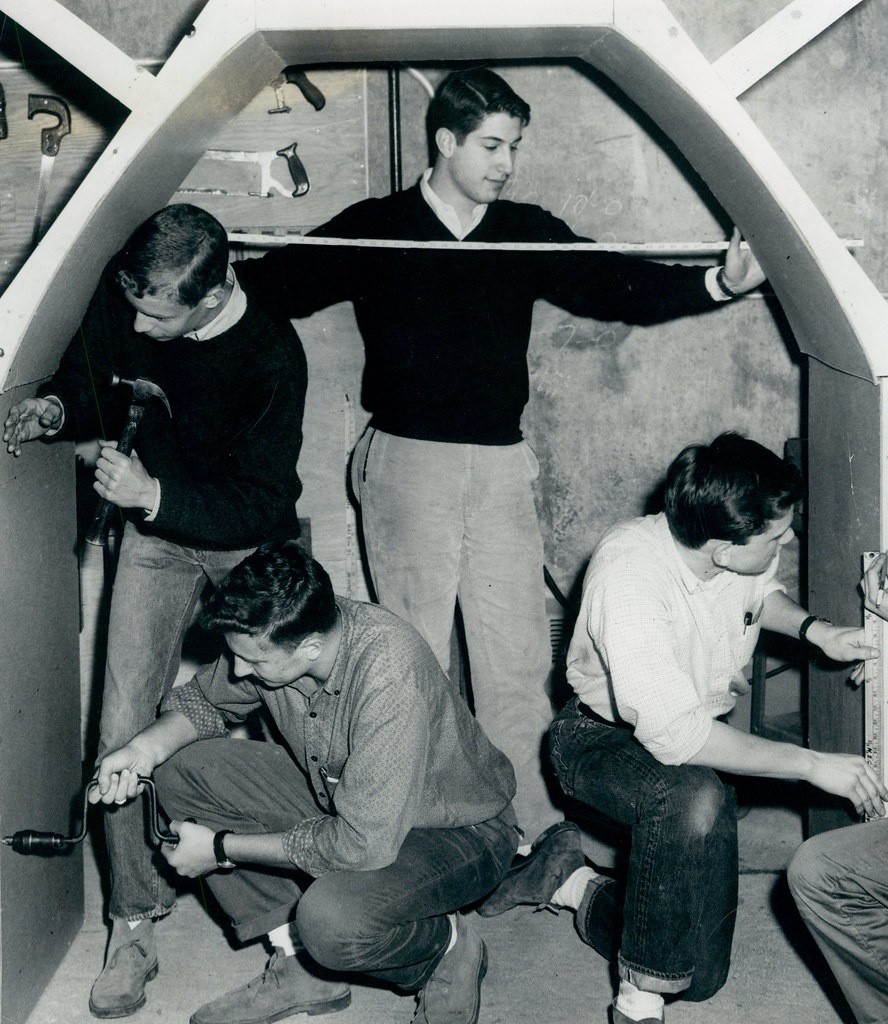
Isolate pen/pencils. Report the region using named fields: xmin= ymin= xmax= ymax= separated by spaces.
xmin=876 ymin=562 xmax=888 ymax=607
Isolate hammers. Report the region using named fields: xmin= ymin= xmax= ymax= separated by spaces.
xmin=82 ymin=373 xmax=172 ymax=545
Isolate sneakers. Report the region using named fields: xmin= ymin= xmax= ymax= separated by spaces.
xmin=409 ymin=909 xmax=488 ymax=1024
xmin=190 ymin=942 xmax=351 ymax=1023
xmin=88 ymin=915 xmax=159 ymax=1018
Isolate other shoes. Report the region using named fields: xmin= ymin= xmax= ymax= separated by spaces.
xmin=477 ymin=820 xmax=584 ymax=917
xmin=611 ymin=997 xmax=667 ymax=1024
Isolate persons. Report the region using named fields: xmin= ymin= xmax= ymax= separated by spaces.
xmin=4 ymin=202 xmax=310 ymax=1018
xmin=477 ymin=433 xmax=888 ymax=1024
xmin=231 ymin=68 xmax=768 ymax=865
xmin=786 ymin=821 xmax=888 ymax=1024
xmin=89 ymin=540 xmax=520 ymax=1024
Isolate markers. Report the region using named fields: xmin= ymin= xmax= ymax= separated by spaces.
xmin=743 ymin=611 xmax=753 ymax=635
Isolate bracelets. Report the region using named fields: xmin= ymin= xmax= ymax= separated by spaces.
xmin=716 ymin=269 xmax=737 ymax=297
xmin=798 ymin=614 xmax=829 ymax=643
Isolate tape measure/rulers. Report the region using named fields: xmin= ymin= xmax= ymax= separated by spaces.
xmin=864 ymin=551 xmax=885 ymax=821
